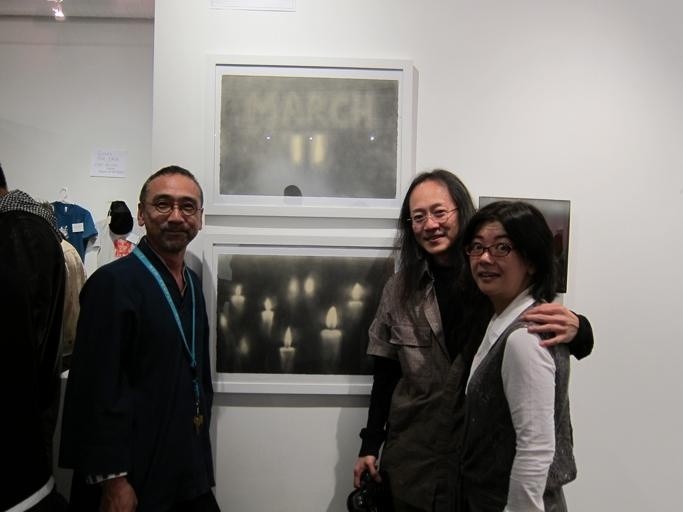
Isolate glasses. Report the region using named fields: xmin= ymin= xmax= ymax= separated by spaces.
xmin=139 ymin=198 xmax=203 ymax=217
xmin=404 ymin=206 xmax=462 ymax=226
xmin=463 ymin=241 xmax=519 ymax=258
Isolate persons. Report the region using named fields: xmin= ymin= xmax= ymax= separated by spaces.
xmin=452 ymin=200 xmax=578 ymax=511
xmin=55 ymin=164 xmax=226 ymax=512
xmin=1 ymin=165 xmax=67 ymax=510
xmin=351 ymin=167 xmax=597 ymax=511
xmin=38 ymin=200 xmax=86 ymax=373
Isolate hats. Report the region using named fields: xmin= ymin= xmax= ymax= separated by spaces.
xmin=107 ymin=200 xmax=133 ymax=235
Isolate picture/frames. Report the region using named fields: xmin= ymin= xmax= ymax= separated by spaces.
xmin=203 ymin=232 xmax=404 ymax=398
xmin=202 ymin=52 xmax=416 ymax=222
